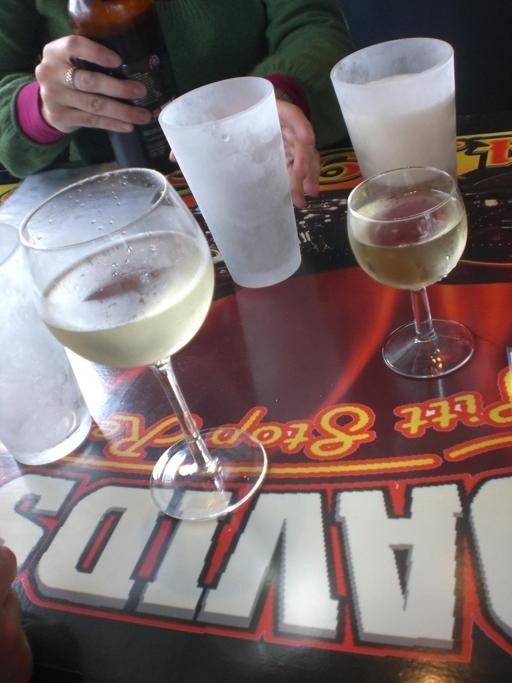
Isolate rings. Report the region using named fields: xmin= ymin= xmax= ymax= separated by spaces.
xmin=66 ymin=63 xmax=77 ymax=89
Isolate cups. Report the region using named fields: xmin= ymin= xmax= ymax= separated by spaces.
xmin=2 ymin=221 xmax=91 ymax=468
xmin=329 ymin=36 xmax=456 ymax=182
xmin=158 ymin=73 xmax=302 ymax=289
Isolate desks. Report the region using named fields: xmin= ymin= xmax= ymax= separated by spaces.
xmin=0 ymin=111 xmax=512 ymax=683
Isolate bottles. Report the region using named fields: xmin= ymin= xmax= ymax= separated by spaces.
xmin=66 ymin=0 xmax=178 ymax=173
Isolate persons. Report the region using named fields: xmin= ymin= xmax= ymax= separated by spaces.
xmin=1 ymin=543 xmax=38 ymax=681
xmin=0 ymin=0 xmax=357 ymax=212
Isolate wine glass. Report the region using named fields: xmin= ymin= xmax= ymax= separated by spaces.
xmin=17 ymin=166 xmax=268 ymax=521
xmin=349 ymin=164 xmax=475 ymax=376
